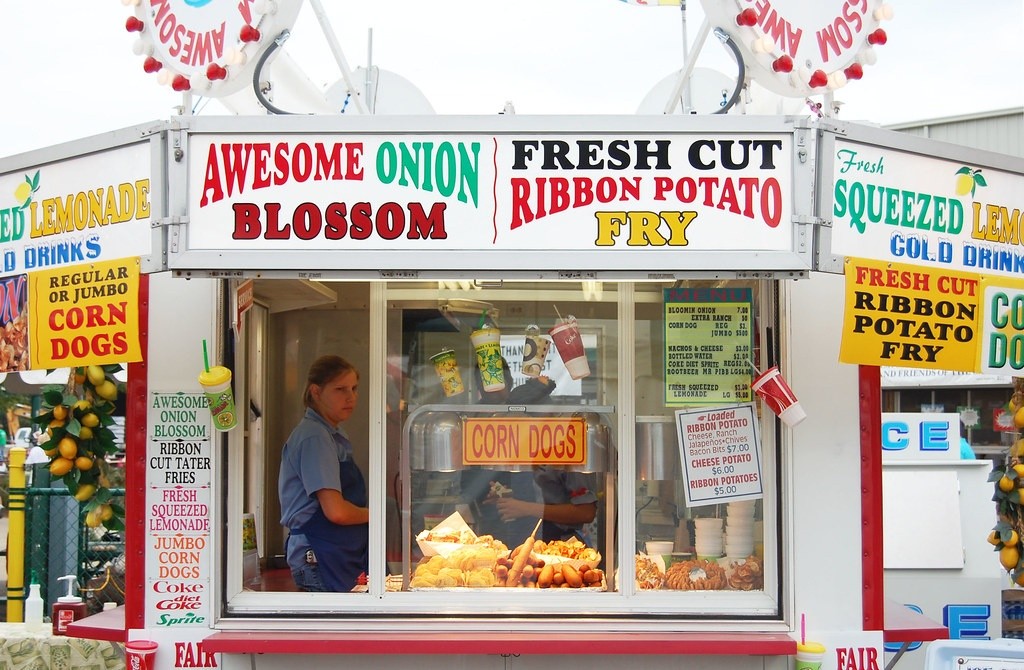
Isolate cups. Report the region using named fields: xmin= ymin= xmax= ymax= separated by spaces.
xmin=752 ymin=368 xmax=806 ymax=427
xmin=645 ymin=541 xmax=674 ymax=569
xmin=200 ymin=365 xmax=238 ymax=432
xmin=424 ymin=515 xmax=448 ymax=529
xmin=795 ymin=643 xmax=826 ymax=670
xmin=521 ymin=335 xmax=551 ymax=378
xmin=470 ymin=328 xmax=505 ymax=392
xmin=725 ymin=499 xmax=755 ymax=561
xmin=429 ymin=350 xmax=466 ymax=397
xmin=549 ymin=321 xmax=591 ymax=379
xmin=242 ymin=514 xmax=256 ymax=551
xmin=694 ymin=518 xmax=724 ymax=563
xmin=126 ymin=640 xmax=158 ymax=670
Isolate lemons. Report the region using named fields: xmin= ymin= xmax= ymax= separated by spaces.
xmin=45 ymin=365 xmax=117 ymax=528
xmin=987 ymin=399 xmax=1024 ymax=587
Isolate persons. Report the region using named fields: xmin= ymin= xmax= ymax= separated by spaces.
xmin=457 ymin=353 xmax=599 ymax=552
xmin=278 ymin=355 xmax=369 ymax=592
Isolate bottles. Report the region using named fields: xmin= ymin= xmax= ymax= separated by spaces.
xmin=25 ymin=584 xmax=44 ymax=629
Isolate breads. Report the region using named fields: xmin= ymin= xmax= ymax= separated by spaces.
xmin=665 ymin=559 xmax=727 ymax=590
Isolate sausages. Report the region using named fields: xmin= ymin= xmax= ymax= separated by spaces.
xmin=498 ymin=539 xmax=603 ymax=588
